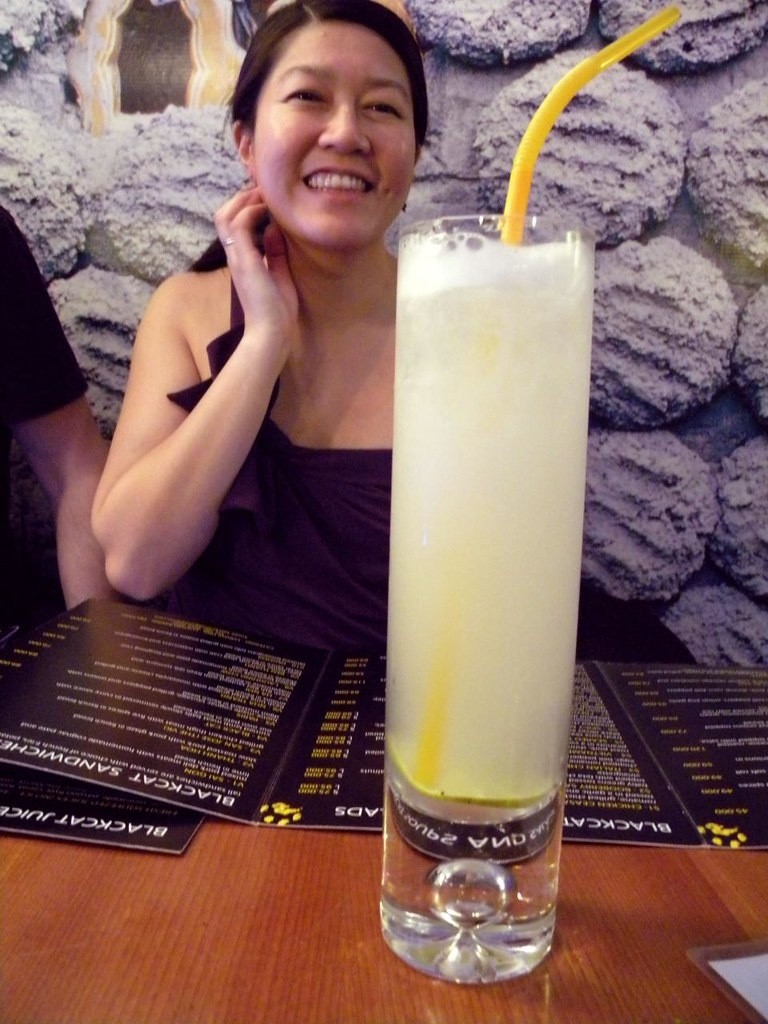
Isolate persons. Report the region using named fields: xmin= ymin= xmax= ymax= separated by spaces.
xmin=0 ymin=207 xmax=106 ymax=650
xmin=91 ymin=1 xmax=429 ymax=662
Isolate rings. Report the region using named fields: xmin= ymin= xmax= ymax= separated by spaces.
xmin=225 ymin=237 xmax=237 ymax=245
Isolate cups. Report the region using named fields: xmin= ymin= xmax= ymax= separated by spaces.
xmin=377 ymin=210 xmax=594 ymax=985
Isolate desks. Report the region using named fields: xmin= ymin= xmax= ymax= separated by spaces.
xmin=0 ymin=814 xmax=768 ymax=1024
